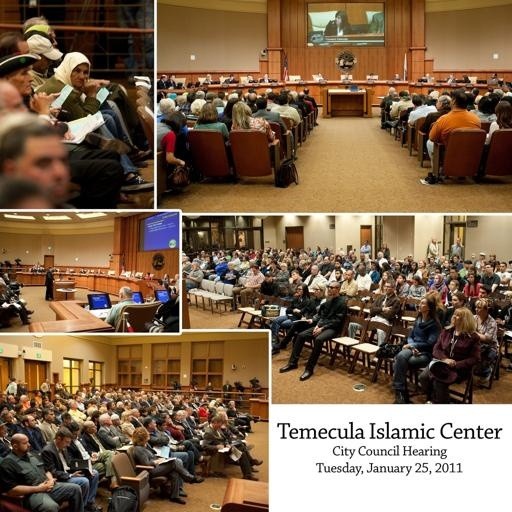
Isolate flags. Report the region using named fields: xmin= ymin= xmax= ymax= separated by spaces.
xmin=403 ymin=52 xmax=410 ymax=72
xmin=280 ymin=52 xmax=292 ymax=81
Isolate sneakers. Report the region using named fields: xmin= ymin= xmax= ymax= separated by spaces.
xmin=129 ymin=146 xmax=153 ymax=163
xmin=14 ymin=308 xmax=35 ymax=325
xmin=117 ymin=173 xmax=155 ymax=193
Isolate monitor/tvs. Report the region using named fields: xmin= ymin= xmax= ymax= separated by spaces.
xmin=154 ymin=290 xmax=171 ymax=303
xmin=132 ymin=292 xmax=144 ymax=303
xmin=88 ymin=294 xmax=111 ymax=310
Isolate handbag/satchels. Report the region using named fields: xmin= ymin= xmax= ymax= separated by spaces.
xmin=167 ymin=164 xmax=192 ymax=187
xmin=275 ymin=158 xmax=300 ymax=188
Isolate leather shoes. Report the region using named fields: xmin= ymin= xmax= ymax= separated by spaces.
xmin=167 ymin=452 xmax=264 ymax=506
xmin=298 ymin=367 xmax=315 ymax=381
xmin=278 ymin=361 xmax=298 ymax=374
xmin=88 ymin=479 xmax=121 ymax=512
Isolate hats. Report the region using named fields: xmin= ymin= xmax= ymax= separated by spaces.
xmin=425 ymin=358 xmax=459 ymax=385
xmin=25 ymin=33 xmax=64 ymax=62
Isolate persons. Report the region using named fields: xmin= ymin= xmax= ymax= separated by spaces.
xmin=380 ymin=71 xmax=512 ymax=186
xmin=368 ymin=9 xmax=385 ymax=35
xmin=158 ymin=66 xmax=318 ymax=189
xmin=156 ymin=279 xmax=179 ymax=322
xmin=102 ymin=287 xmax=138 ymax=329
xmin=0 ymin=12 xmax=152 ymax=209
xmin=0 ymin=272 xmax=35 ymax=326
xmin=323 ymin=8 xmax=355 ymax=37
xmin=28 ymin=264 xmax=179 ymax=286
xmin=44 ymin=266 xmax=54 ymax=302
xmin=339 ymin=72 xmax=355 ymax=81
xmin=316 ymin=70 xmax=324 ymax=81
xmin=0 ymin=373 xmax=264 ymax=512
xmin=366 ymin=71 xmax=378 ymax=80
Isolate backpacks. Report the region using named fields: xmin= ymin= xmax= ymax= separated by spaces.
xmin=106 ymin=484 xmax=139 ymax=512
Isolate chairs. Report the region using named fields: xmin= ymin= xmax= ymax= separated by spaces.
xmin=158 ymin=99 xmax=317 ymax=204
xmin=1 ymin=445 xmax=209 ymax=512
xmin=380 ymin=96 xmax=512 ymax=186
xmin=186 ymin=278 xmax=512 ymax=405
